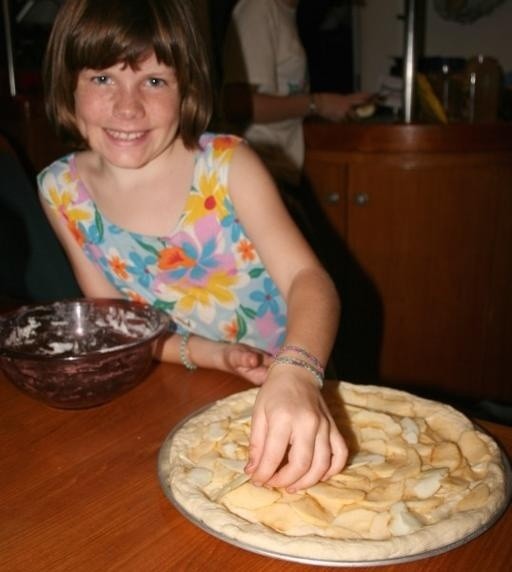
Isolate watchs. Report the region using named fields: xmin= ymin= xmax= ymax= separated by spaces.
xmin=309 ymin=93 xmax=316 ymax=115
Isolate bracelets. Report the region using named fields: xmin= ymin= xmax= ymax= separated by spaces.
xmin=268 ymin=358 xmax=324 ymax=390
xmin=271 ymin=345 xmax=326 ymax=375
xmin=180 ymin=332 xmax=198 ymax=370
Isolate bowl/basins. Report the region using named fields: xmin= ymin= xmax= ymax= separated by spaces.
xmin=0 ymin=296 xmax=172 ymax=411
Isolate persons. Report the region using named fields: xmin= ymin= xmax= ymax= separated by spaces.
xmin=214 ymin=0 xmax=368 ymax=193
xmin=34 ymin=1 xmax=351 ymax=492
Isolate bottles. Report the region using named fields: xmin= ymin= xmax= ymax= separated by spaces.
xmin=445 ymin=54 xmax=504 ymax=128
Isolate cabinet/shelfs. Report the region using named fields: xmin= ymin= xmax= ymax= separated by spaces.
xmin=288 ymin=147 xmax=510 ymax=399
